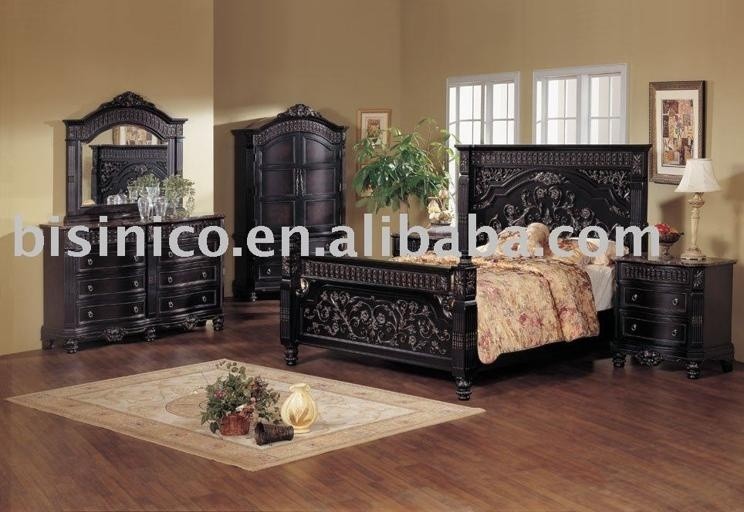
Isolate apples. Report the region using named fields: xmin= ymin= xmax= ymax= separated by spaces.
xmin=654 ymin=223 xmax=670 ymax=234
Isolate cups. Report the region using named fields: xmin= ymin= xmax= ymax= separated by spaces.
xmin=254 ymin=421 xmax=295 ymax=445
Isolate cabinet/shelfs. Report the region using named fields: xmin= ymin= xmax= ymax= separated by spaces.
xmin=231 ymin=102 xmax=350 ymax=303
xmin=42 ymin=211 xmax=224 ymax=353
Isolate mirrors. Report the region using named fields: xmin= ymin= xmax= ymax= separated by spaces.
xmin=62 ymin=90 xmax=186 ymax=221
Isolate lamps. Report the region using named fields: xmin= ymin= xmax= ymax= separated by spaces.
xmin=674 ymin=158 xmax=723 ymax=259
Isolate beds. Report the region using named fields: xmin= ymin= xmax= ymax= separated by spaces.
xmin=279 ymin=145 xmax=651 ymax=402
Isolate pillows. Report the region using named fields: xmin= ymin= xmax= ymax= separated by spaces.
xmin=489 ymin=226 xmax=628 ymax=266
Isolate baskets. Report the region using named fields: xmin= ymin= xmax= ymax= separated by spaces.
xmin=216 ymin=413 xmax=249 ymax=436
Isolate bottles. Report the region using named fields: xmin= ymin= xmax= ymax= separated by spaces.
xmin=107 ymin=194 xmax=127 ymax=205
xmin=137 ymin=191 xmax=168 ymax=222
xmin=282 ymin=384 xmax=318 ymax=435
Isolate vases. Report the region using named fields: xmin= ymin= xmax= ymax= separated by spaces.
xmin=254 ymin=383 xmax=316 ymax=446
xmin=219 ymin=412 xmax=251 ymax=436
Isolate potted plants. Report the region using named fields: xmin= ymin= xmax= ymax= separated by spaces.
xmin=129 ymin=174 xmax=195 ymax=218
xmin=349 ymin=118 xmax=461 ymax=236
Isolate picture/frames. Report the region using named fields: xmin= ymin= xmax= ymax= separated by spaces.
xmin=353 ymin=108 xmax=392 ymax=202
xmin=647 ymin=80 xmax=705 ymax=185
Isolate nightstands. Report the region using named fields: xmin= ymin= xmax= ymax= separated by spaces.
xmin=610 ymin=254 xmax=735 ymax=379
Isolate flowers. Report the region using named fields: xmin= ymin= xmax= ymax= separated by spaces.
xmin=198 ymin=361 xmax=282 ymax=440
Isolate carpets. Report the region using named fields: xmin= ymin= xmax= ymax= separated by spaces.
xmin=2 ymin=358 xmax=486 ymax=471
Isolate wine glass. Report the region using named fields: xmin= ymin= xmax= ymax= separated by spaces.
xmin=126 ymin=186 xmax=143 ymax=204
xmin=147 ymin=187 xmax=161 ymax=219
